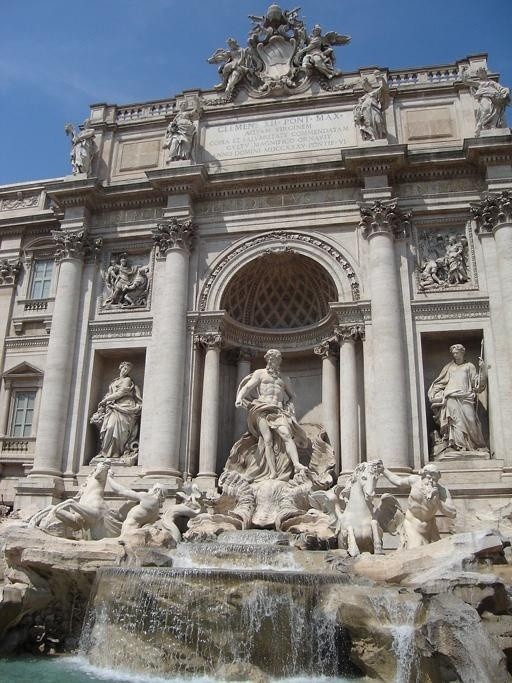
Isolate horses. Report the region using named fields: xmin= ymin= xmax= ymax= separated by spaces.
xmin=28 ymin=459 xmax=122 ymax=541
xmin=339 ymin=460 xmax=383 ymax=557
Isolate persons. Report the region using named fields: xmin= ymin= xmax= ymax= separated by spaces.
xmin=463 ymin=63 xmax=511 ymax=133
xmin=420 ymin=236 xmax=468 ymax=290
xmin=213 ymin=38 xmax=247 ymax=94
xmin=72 ymin=119 xmax=95 ymax=173
xmin=354 ymin=71 xmax=384 ymax=141
xmin=235 ymin=349 xmax=308 ymax=480
xmin=167 ymin=98 xmax=200 ymax=162
xmin=102 ymin=258 xmax=148 ymax=307
xmin=382 ymin=464 xmax=457 ymax=549
xmin=107 ymin=469 xmax=168 ymax=535
xmin=427 ymin=344 xmax=488 ymax=451
xmin=296 ymin=24 xmax=333 ymax=79
xmin=90 ymin=362 xmax=142 ymax=458
